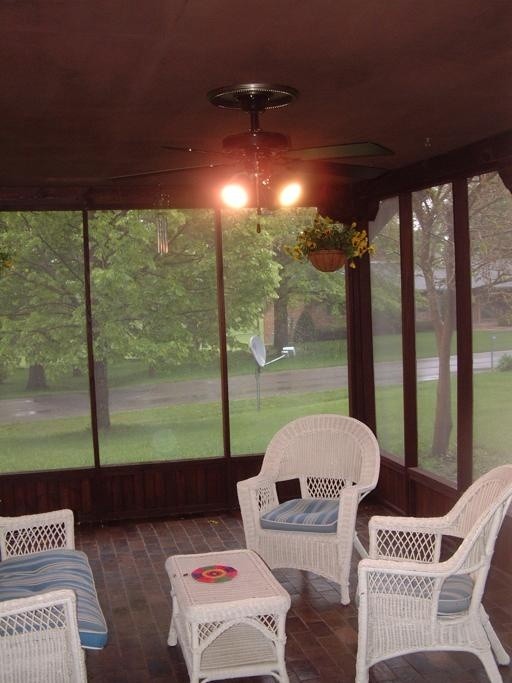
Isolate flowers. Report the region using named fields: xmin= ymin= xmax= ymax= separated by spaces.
xmin=282 ymin=216 xmax=375 ymax=269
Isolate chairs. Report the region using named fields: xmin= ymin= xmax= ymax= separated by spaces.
xmin=238 ymin=414 xmax=379 ymax=605
xmin=0 ymin=509 xmax=89 ymax=682
xmin=356 ymin=462 xmax=512 ymax=683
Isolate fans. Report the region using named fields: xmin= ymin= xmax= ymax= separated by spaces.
xmin=91 ymin=81 xmax=394 ymax=185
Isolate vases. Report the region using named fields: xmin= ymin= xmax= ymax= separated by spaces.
xmin=308 ymin=249 xmax=349 ymax=272
